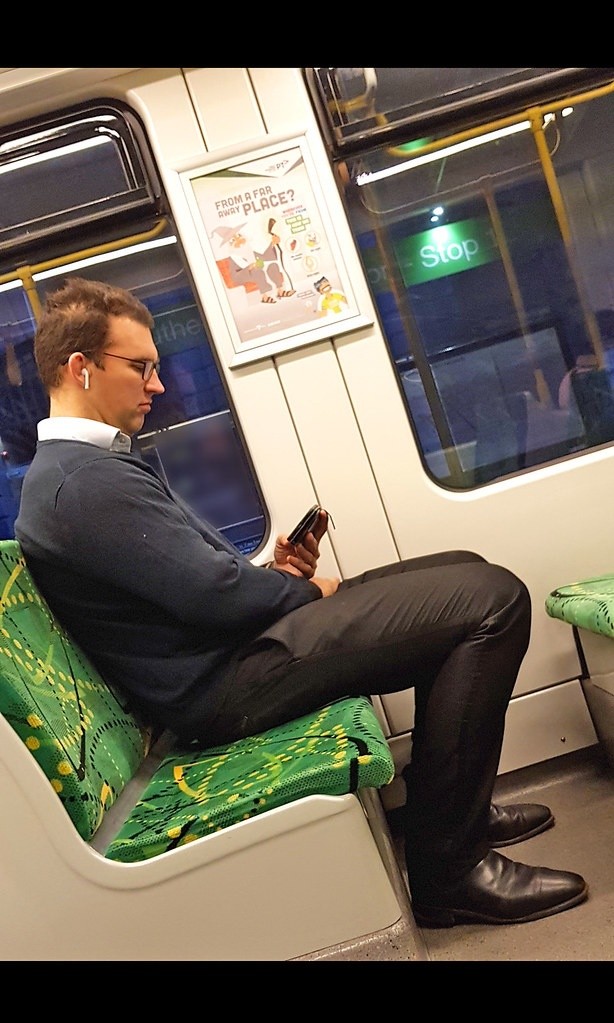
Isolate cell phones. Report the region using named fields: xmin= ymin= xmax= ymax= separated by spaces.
xmin=288 ymin=505 xmax=322 ymax=546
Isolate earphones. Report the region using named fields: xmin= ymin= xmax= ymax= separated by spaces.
xmin=82 ymin=368 xmax=89 ymax=389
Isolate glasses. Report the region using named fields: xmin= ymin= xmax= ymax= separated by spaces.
xmin=62 ymin=350 xmax=161 ymax=382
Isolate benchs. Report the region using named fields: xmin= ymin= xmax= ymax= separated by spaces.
xmin=0 ymin=538 xmax=396 ymax=889
xmin=546 ymin=569 xmax=613 ymax=644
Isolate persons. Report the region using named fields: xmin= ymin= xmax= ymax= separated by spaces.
xmin=13 ymin=280 xmax=589 ymax=928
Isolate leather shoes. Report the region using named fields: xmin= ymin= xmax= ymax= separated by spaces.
xmin=489 ymin=803 xmax=556 ymax=848
xmin=411 ymin=848 xmax=590 ymax=928
